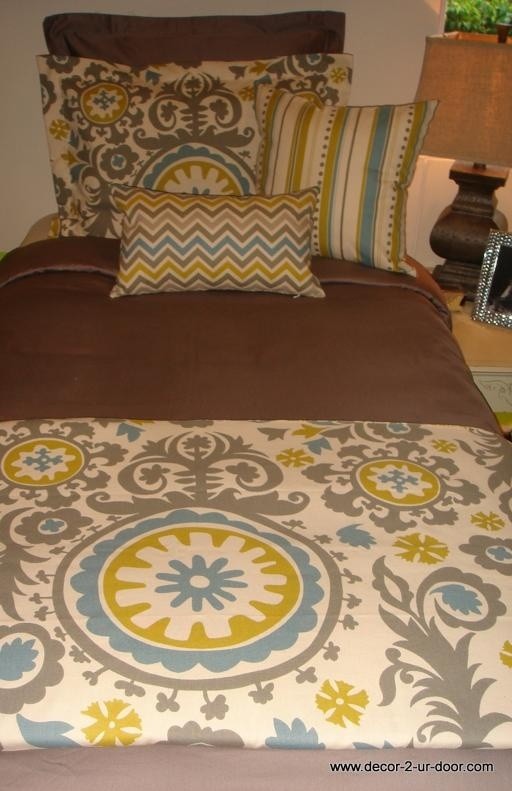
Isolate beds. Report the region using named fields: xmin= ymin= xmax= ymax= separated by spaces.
xmin=0 ymin=202 xmax=512 ymax=788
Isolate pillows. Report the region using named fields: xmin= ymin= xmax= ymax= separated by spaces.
xmin=34 ymin=12 xmax=442 ymax=300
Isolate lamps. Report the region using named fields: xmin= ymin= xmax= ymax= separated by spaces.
xmin=406 ymin=30 xmax=512 ymax=295
